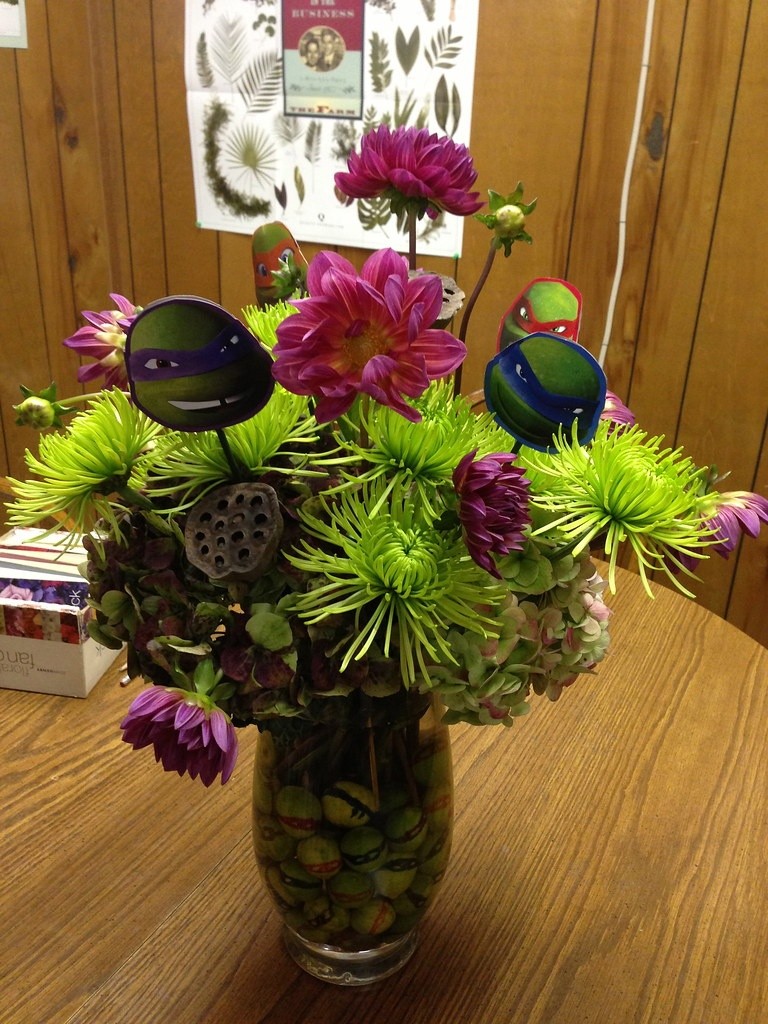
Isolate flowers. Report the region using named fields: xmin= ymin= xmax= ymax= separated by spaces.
xmin=4 ymin=124 xmax=767 ymax=787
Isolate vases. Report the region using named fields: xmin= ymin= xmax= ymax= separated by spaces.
xmin=253 ymin=690 xmax=454 ymax=986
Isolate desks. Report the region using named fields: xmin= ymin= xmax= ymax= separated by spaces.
xmin=1 ymin=555 xmax=767 ymax=1024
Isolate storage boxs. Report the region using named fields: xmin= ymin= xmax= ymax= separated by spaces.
xmin=0 ymin=526 xmax=127 ymax=699
